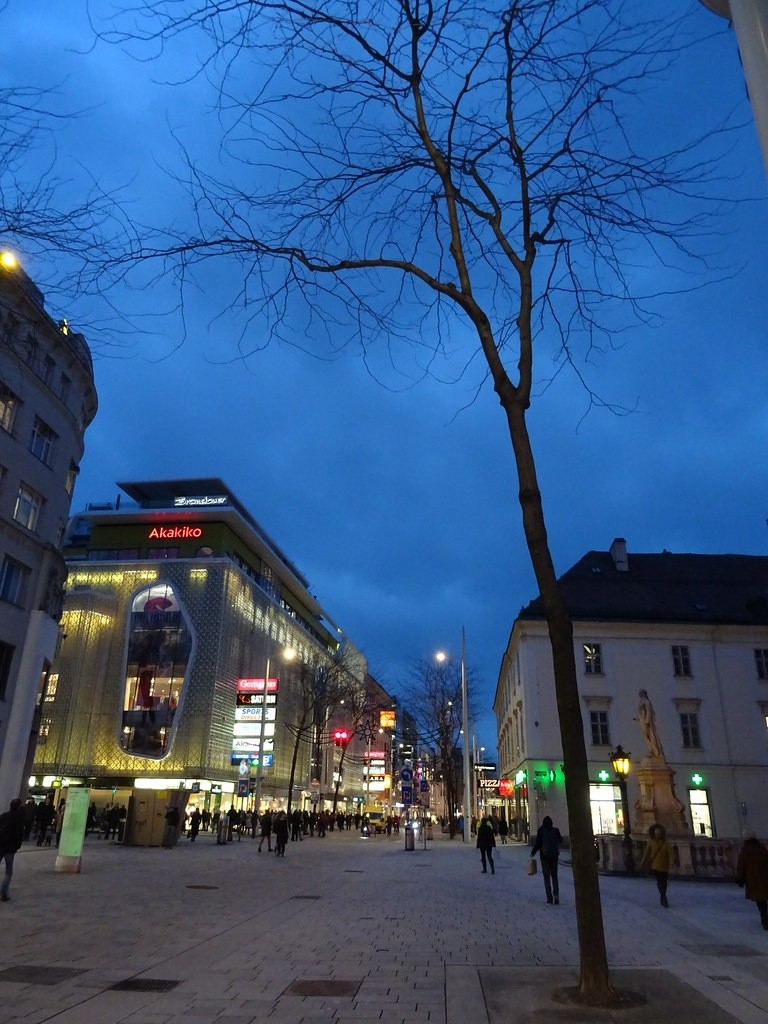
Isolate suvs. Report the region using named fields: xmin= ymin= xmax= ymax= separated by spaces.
xmin=363 ymin=811 xmax=386 ymax=834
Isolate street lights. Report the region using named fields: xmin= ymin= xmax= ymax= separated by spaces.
xmin=254 ymin=648 xmax=295 ymax=811
xmin=366 ymin=728 xmax=383 ymax=805
xmin=436 ymin=653 xmax=472 ymax=844
xmin=608 ymin=745 xmax=638 ymax=877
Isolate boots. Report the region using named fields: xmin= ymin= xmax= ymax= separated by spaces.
xmin=480 ymin=859 xmax=487 ymax=873
xmin=657 ymin=883 xmax=669 ymax=908
xmin=490 ymin=859 xmax=495 ymax=874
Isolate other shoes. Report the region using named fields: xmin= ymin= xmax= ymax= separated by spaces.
xmin=554 ymin=897 xmax=559 ymax=905
xmin=547 ymin=899 xmax=553 ymax=904
xmin=258 ymin=848 xmax=261 ymax=852
xmin=268 ymin=849 xmax=273 ymax=852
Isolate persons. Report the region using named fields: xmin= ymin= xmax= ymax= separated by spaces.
xmin=736 ymin=829 xmax=768 ymax=930
xmin=0 ymin=796 xmax=563 ymax=906
xmin=641 ymin=824 xmax=676 ymax=908
xmin=633 ymin=689 xmax=660 ymax=759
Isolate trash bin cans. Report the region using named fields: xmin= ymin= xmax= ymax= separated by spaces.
xmin=216 ymin=815 xmax=230 ymax=844
xmin=405 ymin=824 xmax=413 ymax=851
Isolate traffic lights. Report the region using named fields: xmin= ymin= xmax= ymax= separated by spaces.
xmin=335 ymin=729 xmax=347 ymax=747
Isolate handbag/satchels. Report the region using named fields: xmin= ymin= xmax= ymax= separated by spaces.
xmin=644 ymin=863 xmax=653 ymax=874
xmin=527 ymin=856 xmax=537 ymax=876
xmin=187 ymin=831 xmax=190 ymax=838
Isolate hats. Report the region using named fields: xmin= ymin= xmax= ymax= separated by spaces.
xmin=743 ymin=829 xmax=756 ymax=840
